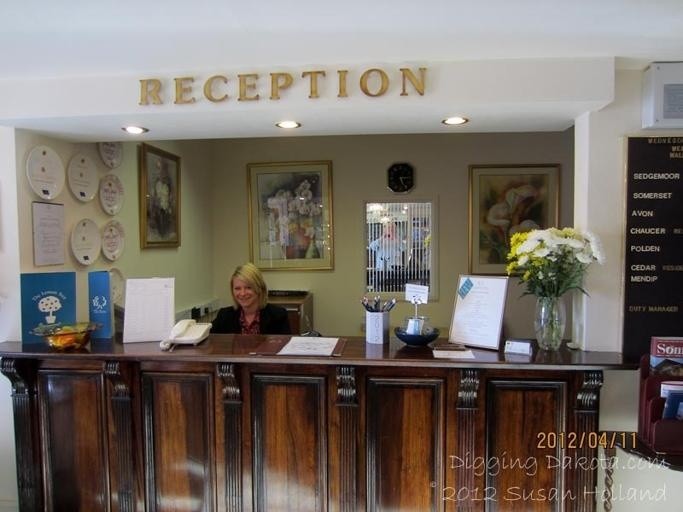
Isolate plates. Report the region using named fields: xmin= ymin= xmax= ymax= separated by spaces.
xmin=98 ymin=175 xmax=125 ymax=216
xmin=66 ymin=153 xmax=99 ymax=204
xmin=106 ymin=268 xmax=125 ymax=304
xmin=25 ymin=145 xmax=66 ymax=199
xmin=101 ymin=220 xmax=125 ymax=261
xmin=98 ymin=142 xmax=122 ymax=169
xmin=69 ymin=218 xmax=102 ymax=265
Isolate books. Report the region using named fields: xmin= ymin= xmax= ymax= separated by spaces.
xmin=649 ymin=336 xmax=683 ymax=420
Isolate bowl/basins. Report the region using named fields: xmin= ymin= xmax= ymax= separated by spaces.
xmin=31 ymin=322 xmax=101 ymax=350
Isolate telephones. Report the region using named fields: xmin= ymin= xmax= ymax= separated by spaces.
xmin=159 ymin=319 xmax=212 ymax=353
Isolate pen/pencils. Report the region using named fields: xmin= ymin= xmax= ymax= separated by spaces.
xmin=359 ymin=295 xmax=396 ymax=312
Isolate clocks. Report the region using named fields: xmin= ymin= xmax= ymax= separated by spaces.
xmin=385 ymin=161 xmax=417 ymax=195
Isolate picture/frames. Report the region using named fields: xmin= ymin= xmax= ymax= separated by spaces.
xmin=140 ymin=143 xmax=182 ymax=249
xmin=468 ymin=162 xmax=562 ymax=277
xmin=361 ymin=192 xmax=439 ymax=303
xmin=246 ymin=160 xmax=335 ymax=272
xmin=448 ymin=273 xmax=508 ymax=350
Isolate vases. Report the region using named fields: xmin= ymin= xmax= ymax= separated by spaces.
xmin=535 ymin=297 xmax=567 ymax=352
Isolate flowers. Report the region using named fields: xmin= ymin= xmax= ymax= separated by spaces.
xmin=506 ymin=226 xmax=607 ymax=347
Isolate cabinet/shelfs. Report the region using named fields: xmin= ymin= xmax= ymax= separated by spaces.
xmin=264 ymin=291 xmax=313 ymax=336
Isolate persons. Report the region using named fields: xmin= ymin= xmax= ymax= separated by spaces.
xmin=210 ymin=263 xmax=291 ymax=335
xmin=369 ymin=223 xmax=412 ymax=270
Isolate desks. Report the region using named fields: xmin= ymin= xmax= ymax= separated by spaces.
xmin=599 ymin=431 xmax=683 ymax=512
